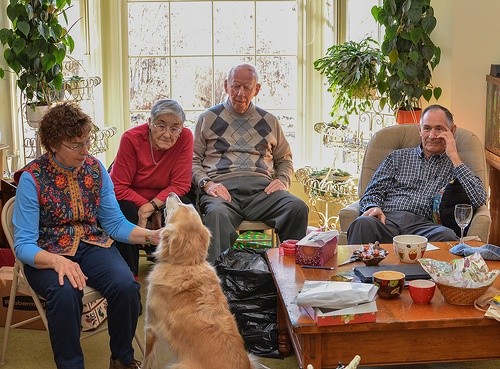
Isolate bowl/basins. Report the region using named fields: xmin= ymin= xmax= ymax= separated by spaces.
xmin=393 ymin=235 xmax=428 ymax=263
xmin=408 ymin=280 xmax=436 ymax=305
xmin=372 ymin=270 xmax=405 ymax=300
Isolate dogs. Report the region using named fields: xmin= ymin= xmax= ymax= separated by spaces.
xmin=142 ymin=190 xmax=250 ymax=369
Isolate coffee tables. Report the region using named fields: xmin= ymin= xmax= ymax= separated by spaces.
xmin=265 ymin=246 xmax=500 ymax=369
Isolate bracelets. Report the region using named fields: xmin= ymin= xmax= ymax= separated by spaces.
xmin=146 ymin=229 xmax=150 ymax=244
xmin=149 ymin=200 xmax=159 ymax=213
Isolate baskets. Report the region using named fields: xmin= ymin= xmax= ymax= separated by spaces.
xmin=433 ymin=270 xmax=499 ymax=306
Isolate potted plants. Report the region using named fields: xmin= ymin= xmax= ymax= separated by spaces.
xmin=369 ymin=0 xmax=442 ymax=123
xmin=0 ymin=0 xmax=83 ymax=122
xmin=312 ymin=38 xmax=386 ymax=126
xmin=311 ymin=168 xmax=351 ymax=200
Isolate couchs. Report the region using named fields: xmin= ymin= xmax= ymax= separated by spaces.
xmin=339 ymin=124 xmax=491 ymax=244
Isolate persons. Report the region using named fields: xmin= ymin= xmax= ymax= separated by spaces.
xmin=109 ymin=99 xmax=194 ymax=315
xmin=348 ymin=104 xmax=487 ymax=244
xmin=12 ymin=100 xmax=163 ymax=369
xmin=191 ymin=64 xmax=308 ymax=262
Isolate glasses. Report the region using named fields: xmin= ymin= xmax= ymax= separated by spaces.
xmin=152 ymin=120 xmax=182 ymax=133
xmin=59 ymin=134 xmax=96 ymax=151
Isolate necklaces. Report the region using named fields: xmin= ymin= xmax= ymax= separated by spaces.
xmin=149 ymin=132 xmax=160 ymax=166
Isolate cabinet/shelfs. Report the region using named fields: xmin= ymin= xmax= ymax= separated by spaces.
xmin=20 ymin=56 xmax=118 ymax=165
xmin=295 ymin=89 xmax=423 ymax=231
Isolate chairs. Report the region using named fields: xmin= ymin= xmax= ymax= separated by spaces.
xmin=236 ymin=220 xmax=280 ymax=247
xmin=0 ymin=195 xmax=144 ymax=363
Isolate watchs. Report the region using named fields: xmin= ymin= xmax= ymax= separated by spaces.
xmin=199 ymin=179 xmax=210 ymax=192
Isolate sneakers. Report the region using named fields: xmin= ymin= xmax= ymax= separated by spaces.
xmin=109 ymin=353 xmax=141 ymax=369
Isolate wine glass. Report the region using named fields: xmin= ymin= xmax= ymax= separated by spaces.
xmin=454 ymin=204 xmax=472 ymax=247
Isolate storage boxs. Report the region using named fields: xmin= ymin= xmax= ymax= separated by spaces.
xmin=0 ymin=266 xmax=48 ymax=330
xmin=303 ymin=303 xmax=377 ymax=325
xmin=232 ymin=230 xmax=277 ymax=249
